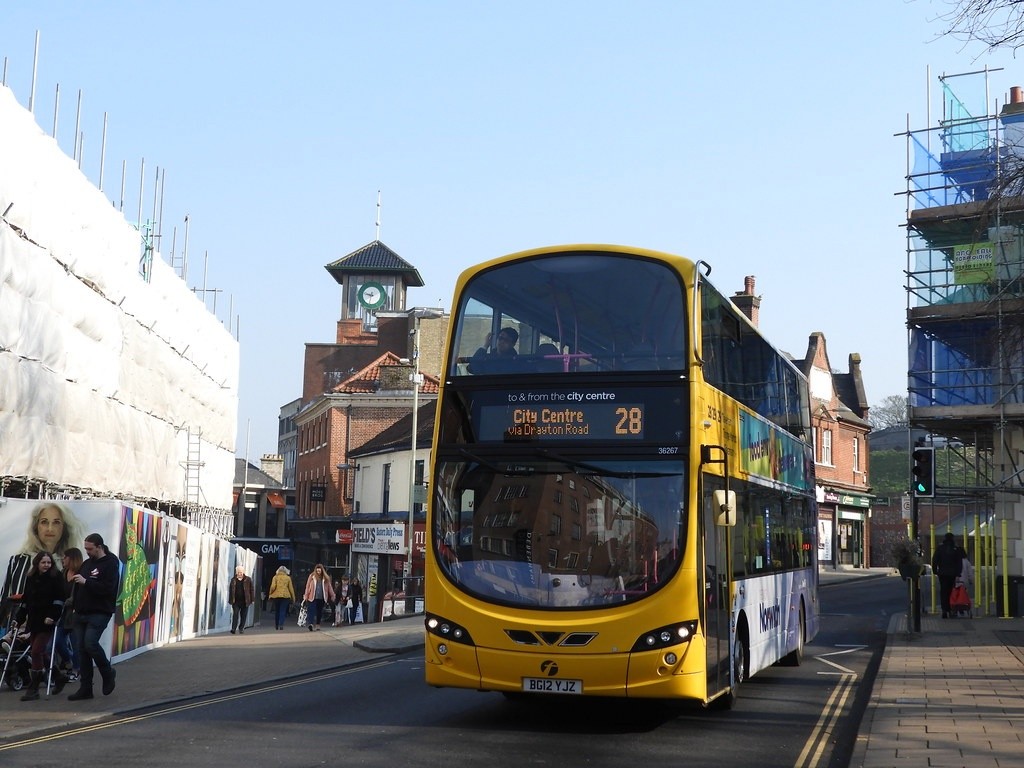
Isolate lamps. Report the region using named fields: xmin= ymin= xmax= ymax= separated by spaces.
xmin=337 ymin=464 xmax=360 ymax=472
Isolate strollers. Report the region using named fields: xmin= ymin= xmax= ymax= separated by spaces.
xmin=0 ymin=594 xmax=55 ymax=691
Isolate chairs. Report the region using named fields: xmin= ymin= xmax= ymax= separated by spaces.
xmin=469 ymin=343 xmax=687 ymax=372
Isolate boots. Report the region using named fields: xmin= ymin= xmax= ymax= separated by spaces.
xmin=48 ymin=664 xmax=70 ymax=695
xmin=20 ymin=669 xmax=45 ymax=701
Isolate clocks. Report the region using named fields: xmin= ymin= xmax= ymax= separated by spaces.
xmin=358 ymin=282 xmax=386 ymax=308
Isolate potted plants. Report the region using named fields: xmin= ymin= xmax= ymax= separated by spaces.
xmin=893 ymin=539 xmax=924 ymax=580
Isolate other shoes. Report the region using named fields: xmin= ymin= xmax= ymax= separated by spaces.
xmin=230 ymin=630 xmax=235 ymax=634
xmin=2 ymin=642 xmax=11 ymax=654
xmin=68 ymin=671 xmax=79 ymax=682
xmin=316 ymin=624 xmax=320 ymax=630
xmin=67 ymin=684 xmax=94 ymax=700
xmin=308 ymin=624 xmax=313 ymax=631
xmin=239 ymin=631 xmax=243 ymax=634
xmin=102 ymin=668 xmax=116 ymax=695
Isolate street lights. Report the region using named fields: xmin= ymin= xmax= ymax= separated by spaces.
xmin=406 ymin=310 xmax=443 ymax=576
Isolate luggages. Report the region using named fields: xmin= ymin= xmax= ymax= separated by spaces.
xmin=949 ymin=581 xmax=972 ymax=620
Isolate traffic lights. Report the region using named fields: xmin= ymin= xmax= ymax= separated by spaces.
xmin=912 ymin=446 xmax=935 ymax=499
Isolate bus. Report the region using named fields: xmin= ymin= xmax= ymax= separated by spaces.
xmin=422 ymin=241 xmax=821 ymax=712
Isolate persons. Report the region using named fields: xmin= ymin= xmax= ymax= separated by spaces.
xmin=0 ymin=533 xmax=83 ymax=702
xmin=327 ymin=575 xmax=363 ymax=627
xmin=932 ymin=533 xmax=975 ymax=619
xmin=68 ymin=533 xmax=119 ymax=700
xmin=228 ymin=566 xmax=255 ymax=634
xmin=269 ymin=566 xmax=295 ymax=630
xmin=303 ymin=564 xmax=335 ymax=631
xmin=168 ymin=524 xmax=188 ymax=638
xmin=642 ymin=454 xmax=680 ymax=548
xmin=0 ymin=503 xmax=88 ymax=634
xmin=466 ymin=328 xmax=518 ymax=376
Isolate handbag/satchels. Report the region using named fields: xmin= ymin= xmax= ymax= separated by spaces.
xmin=297 ymin=599 xmax=308 ymax=627
xmin=344 ymin=599 xmax=353 ymax=608
